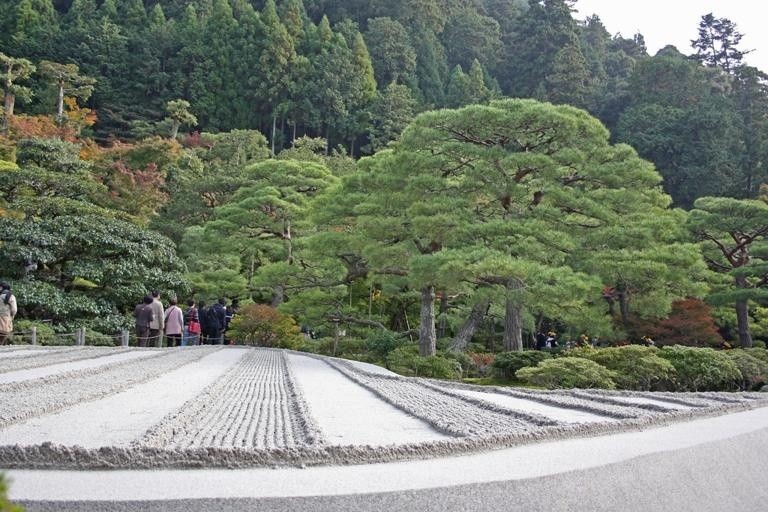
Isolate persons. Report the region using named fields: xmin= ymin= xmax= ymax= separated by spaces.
xmin=149 ymin=288 xmax=165 ymax=348
xmin=163 ymin=296 xmax=185 ymax=347
xmin=224 ymin=298 xmax=239 ymax=345
xmin=207 ymin=297 xmax=227 ymax=344
xmin=134 ymin=295 xmax=156 ymax=347
xmin=1 ymin=282 xmax=17 ymax=346
xmin=533 ymin=330 xmax=655 ymax=350
xmin=182 ymin=299 xmax=201 ymax=346
xmin=198 ymin=300 xmax=210 ymax=345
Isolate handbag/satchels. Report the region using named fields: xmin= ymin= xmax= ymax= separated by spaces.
xmin=188 ymin=309 xmax=201 ymax=335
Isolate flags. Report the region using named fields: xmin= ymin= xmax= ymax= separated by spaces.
xmin=369 ymin=280 xmax=383 ymax=304
